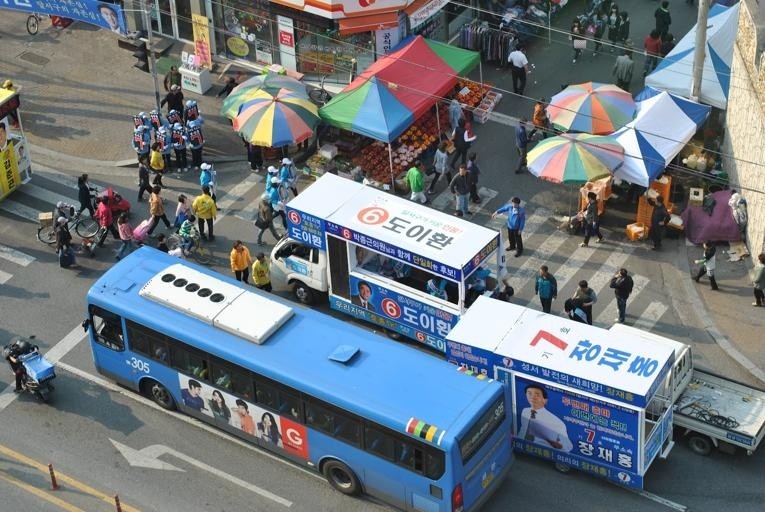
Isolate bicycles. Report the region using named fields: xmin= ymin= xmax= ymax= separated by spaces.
xmin=308 ymin=73 xmax=332 ymax=105
xmin=26 ymin=11 xmax=42 ymax=35
xmin=37 ymin=205 xmax=100 ymax=243
xmin=167 ymin=231 xmax=213 ymax=265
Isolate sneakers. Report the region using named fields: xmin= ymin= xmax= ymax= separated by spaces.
xmin=13 ymin=388 xmax=24 ymax=394
xmin=257 ymin=166 xmax=266 ymax=170
xmin=248 ymin=161 xmax=251 ymax=165
xmin=178 ymin=168 xmax=181 ymax=172
xmin=165 ymin=224 xmax=174 ymax=230
xmin=188 ymin=165 xmax=191 ymax=169
xmin=427 ymin=190 xmax=436 ymax=195
xmin=257 ymin=242 xmax=266 ymax=246
xmin=614 ymin=318 xmax=620 ymax=323
xmin=579 ymin=242 xmax=588 ymax=248
xmin=642 ymin=70 xmax=648 ymax=75
xmin=571 ymin=59 xmax=577 ymax=64
xmin=595 ymin=237 xmax=606 ymax=243
xmin=251 ymin=168 xmax=259 ymax=173
xmin=195 ymin=167 xmax=199 ymax=170
xmin=751 ymin=301 xmax=757 ymax=306
xmin=184 ymin=168 xmax=188 ymax=172
xmin=600 ymin=42 xmax=604 ymax=50
xmin=593 ymin=51 xmax=598 ymax=56
xmin=610 ymin=47 xmax=615 ymax=54
xmin=579 ymin=49 xmax=583 ymax=56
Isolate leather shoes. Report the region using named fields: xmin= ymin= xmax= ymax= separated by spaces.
xmin=158 ymin=184 xmax=167 ymax=189
xmin=137 ymin=198 xmax=144 ymax=202
xmin=115 ymin=256 xmax=120 ymax=261
xmin=514 ymin=251 xmax=523 ymax=257
xmin=515 ymin=169 xmax=524 ymax=174
xmin=505 ymin=246 xmax=517 ymax=251
xmin=691 ymin=276 xmax=699 ymax=282
xmin=216 ymin=207 xmax=221 ymax=211
xmin=651 ymin=240 xmax=663 ymax=251
xmin=202 ymin=235 xmax=207 ymax=242
xmin=209 ymin=234 xmax=215 ymax=241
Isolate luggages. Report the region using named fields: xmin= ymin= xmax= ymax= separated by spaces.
xmin=133 ymin=216 xmax=155 ymax=241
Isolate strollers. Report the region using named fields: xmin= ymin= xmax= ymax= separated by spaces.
xmin=91 ymin=186 xmax=131 ymax=221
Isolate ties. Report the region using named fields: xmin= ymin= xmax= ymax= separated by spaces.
xmin=363 ymin=300 xmax=367 ymax=308
xmin=524 ymin=410 xmax=538 ymax=442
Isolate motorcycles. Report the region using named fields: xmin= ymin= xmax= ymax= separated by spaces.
xmin=1 ymin=334 xmax=56 ymax=404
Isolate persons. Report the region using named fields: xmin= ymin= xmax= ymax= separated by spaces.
xmin=727 ymin=190 xmax=741 ymax=224
xmin=535 ymin=265 xmax=558 ymax=313
xmin=164 ymin=65 xmax=182 ymax=92
xmin=526 ymin=97 xmax=548 ymax=143
xmin=751 ymin=254 xmax=764 ymax=307
xmin=230 ymin=240 xmax=252 ymax=284
xmin=256 ymin=194 xmax=283 ymax=246
xmin=252 ymin=253 xmax=272 ymax=293
xmin=351 ymin=280 xmax=376 ymax=312
xmin=156 ymin=233 xmax=169 ymax=253
xmin=134 ymin=112 xmax=151 ymax=140
xmin=132 ymin=126 xmax=151 ymax=169
xmin=179 ymin=215 xmax=199 ymax=256
xmin=265 ymin=166 xmax=279 ymax=192
xmin=53 ymin=201 xmax=72 ymax=240
xmin=98 ymin=4 xmax=124 ymax=35
xmin=353 ymin=245 xmax=369 ymax=267
xmin=280 ymin=157 xmax=297 ymax=197
xmin=508 ymin=42 xmax=530 ymax=95
xmin=517 ymin=383 xmax=573 ymax=453
xmin=655 ymin=1 xmax=672 ymax=43
xmin=572 ymin=281 xmax=597 ymax=325
xmin=172 ymin=122 xmax=189 ymax=173
xmin=426 ymin=275 xmax=448 ymax=300
xmin=257 ymin=413 xmax=283 ymax=448
xmin=618 ymin=10 xmax=629 ymax=44
xmin=77 ymin=174 xmax=97 ymax=219
xmin=593 ymin=14 xmax=605 ymax=56
xmin=268 ymin=176 xmax=287 ymax=231
xmin=115 ymin=212 xmax=134 ymax=261
xmin=450 ymin=119 xmax=477 ymax=169
xmin=216 ymin=78 xmax=239 ymax=127
xmin=297 ymin=138 xmax=309 ymax=153
xmin=613 ymin=48 xmax=634 ymax=93
xmin=6 ymin=79 xmax=20 ymax=122
xmin=168 ymin=110 xmax=185 ymax=126
xmin=579 ymin=192 xmax=605 ymax=248
xmin=428 ymin=142 xmax=453 ymax=193
xmin=200 ymin=163 xmax=222 ymax=212
xmin=149 ymin=109 xmax=169 ymax=141
xmin=186 ymin=101 xmax=207 ymax=143
xmin=138 ymin=155 xmax=155 ymax=201
xmin=0 ymin=123 xmax=11 ymax=152
xmin=407 ymin=160 xmax=427 ymax=206
xmin=161 ymin=84 xmax=184 ymax=119
xmin=647 ymin=197 xmax=671 ymax=251
xmin=93 ymin=196 xmax=120 ymax=247
xmin=492 ymin=198 xmax=525 ymax=258
xmin=9 ymin=340 xmax=37 ymax=395
xmin=179 ymin=379 xmax=207 ymax=413
xmin=610 ymin=268 xmax=634 ymax=322
xmin=156 ymin=126 xmax=172 ymax=173
xmin=168 ymin=236 xmax=181 ymax=259
xmin=192 ymin=186 xmax=217 ymax=241
xmin=514 ymin=116 xmax=528 ymax=174
xmin=146 ymin=186 xmax=174 ymax=237
xmin=186 ymin=114 xmax=203 ymax=171
xmin=55 ymin=216 xmax=70 ymax=253
xmin=570 ymin=20 xmax=584 ymax=63
xmin=450 ymin=93 xmax=464 ymax=139
xmin=231 ymin=399 xmax=257 ymax=436
xmin=17 ymin=146 xmax=31 ymax=183
xmin=207 ymin=390 xmax=231 ymax=424
xmin=175 ymin=194 xmax=191 ymax=234
xmin=278 ymin=145 xmax=288 ymax=162
xmin=467 ymin=152 xmax=482 ymax=204
xmin=606 ymin=4 xmax=621 ymax=54
xmin=691 ymin=239 xmax=719 ymax=290
xmin=450 ymin=164 xmax=472 ymax=214
xmin=247 ymin=144 xmax=263 ymax=173
xmin=661 ymin=34 xmax=674 ymax=58
xmin=642 ymin=30 xmax=661 ymax=77
xmin=150 ymin=142 xmax=166 ymax=189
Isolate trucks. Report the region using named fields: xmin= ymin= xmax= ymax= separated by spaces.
xmin=610 ymin=323 xmax=765 ymax=457
xmin=267 ymin=171 xmax=502 ymax=355
xmin=438 ymin=295 xmax=675 ymax=493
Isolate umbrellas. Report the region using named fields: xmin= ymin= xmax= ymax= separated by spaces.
xmin=547 ymin=81 xmax=636 ymax=134
xmin=319 ymin=36 xmax=480 ymax=191
xmin=525 ymin=131 xmax=625 ymax=187
xmin=220 ymin=73 xmax=308 ymax=122
xmin=235 ymin=88 xmax=318 ymax=147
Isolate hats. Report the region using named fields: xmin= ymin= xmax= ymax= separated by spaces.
xmin=171 ymin=83 xmax=180 ymax=91
xmin=261 ymin=194 xmax=270 ymax=200
xmin=170 ymin=109 xmax=176 ymax=114
xmin=188 ymin=121 xmax=197 ymax=127
xmin=267 ymin=165 xmax=279 ymax=173
xmin=174 ymin=122 xmax=183 ymax=130
xmin=137 ymin=126 xmax=145 ymax=132
xmin=200 ymin=162 xmax=211 ymax=171
xmin=158 ymin=125 xmax=167 ymax=133
xmin=271 ymin=177 xmax=281 ymax=184
xmin=186 ymin=100 xmax=197 ymax=109
xmin=140 ymin=112 xmax=146 ymax=119
xmin=282 ymin=158 xmax=292 ymax=165
xmin=55 ymin=216 xmax=69 ymax=223
xmin=56 ymin=201 xmax=67 ymax=208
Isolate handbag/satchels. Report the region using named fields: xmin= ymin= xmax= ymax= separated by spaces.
xmin=59 ymin=248 xmax=76 ymax=267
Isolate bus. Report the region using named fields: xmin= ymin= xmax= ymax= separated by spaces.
xmin=83 ymin=245 xmax=515 ymax=511
xmin=0 ymin=88 xmax=34 ymax=202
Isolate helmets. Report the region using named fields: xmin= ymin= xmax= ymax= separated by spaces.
xmin=18 ymin=342 xmax=31 ymax=353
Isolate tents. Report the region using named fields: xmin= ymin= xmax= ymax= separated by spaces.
xmin=642 ymin=1 xmax=739 ymax=110
xmin=607 ymin=87 xmax=712 ymax=188
